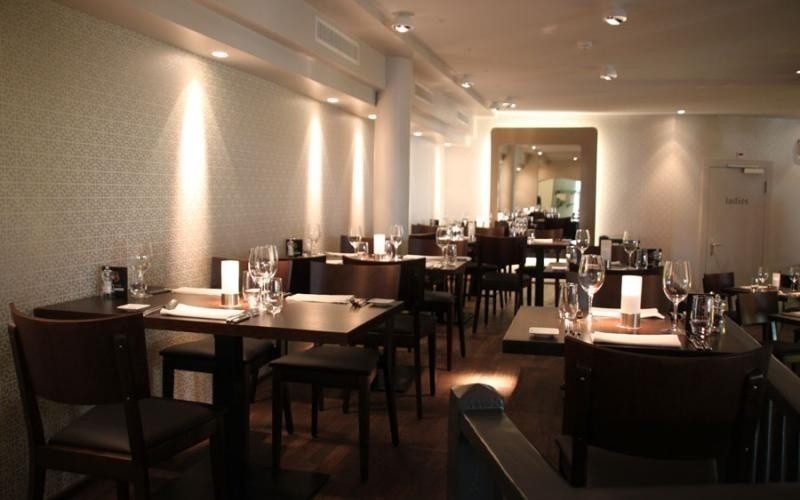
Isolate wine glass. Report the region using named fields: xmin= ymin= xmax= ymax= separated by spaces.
xmin=463 ymin=218 xmax=468 ymax=238
xmin=577 ymin=253 xmax=607 ymax=327
xmin=622 ymin=231 xmax=638 ymax=270
xmin=574 ymin=228 xmax=590 ymax=256
xmin=129 ymin=238 xmax=154 ymax=313
xmin=308 ymin=224 xmax=321 ymax=254
xmin=348 ymin=225 xmax=362 ymax=258
xmin=435 ymin=223 xmax=464 ymax=268
xmin=662 ymin=260 xmax=691 ymax=335
xmin=386 ymin=224 xmax=405 ymax=261
xmin=247 ymin=242 xmax=279 ymax=312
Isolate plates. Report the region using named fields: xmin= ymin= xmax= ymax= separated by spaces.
xmin=117 ymin=302 xmax=151 ymax=313
xmin=368 ymin=298 xmax=394 ymax=307
xmin=530 ymin=326 xmax=561 ymax=338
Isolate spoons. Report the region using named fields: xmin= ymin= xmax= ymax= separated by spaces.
xmin=143 ymin=298 xmax=179 ymax=317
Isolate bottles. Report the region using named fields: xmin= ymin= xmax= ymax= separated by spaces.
xmin=709 ymin=292 xmax=727 ymax=334
xmin=286 ymin=237 xmax=295 ymax=257
xmin=101 ymin=265 xmax=115 ymax=297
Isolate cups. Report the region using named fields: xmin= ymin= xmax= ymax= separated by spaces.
xmin=559 ymin=283 xmax=577 ymax=318
xmin=653 ymin=249 xmax=662 ymax=266
xmin=355 ymin=243 xmax=368 ymax=260
xmin=751 ymin=265 xmax=800 ymax=292
xmin=690 ymin=294 xmax=716 ymax=338
xmin=295 ymin=248 xmax=300 ymax=256
xmin=243 ymin=270 xmax=260 ymax=308
xmin=303 ymin=237 xmax=313 ymax=257
xmin=566 ymin=246 xmax=578 ymax=270
xmin=511 ymin=207 xmax=559 ymax=244
xmin=636 ymin=249 xmax=649 ymax=270
xmin=260 ymin=276 xmax=284 ymax=314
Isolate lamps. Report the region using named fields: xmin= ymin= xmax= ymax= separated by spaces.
xmin=390 ymin=11 xmax=414 ymax=32
xmin=599 ymin=63 xmax=619 ymax=82
xmin=487 ymin=97 xmax=515 ymax=115
xmin=601 ymin=1 xmax=627 ymax=26
xmin=457 ymin=75 xmax=475 ymax=93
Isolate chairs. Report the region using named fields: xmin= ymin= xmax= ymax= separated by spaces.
xmin=341 ymin=255 xmax=454 ymax=396
xmin=496 ymin=209 xmax=578 ymax=229
xmin=701 ymin=271 xmax=735 ymax=316
xmin=562 ymin=336 xmax=770 ymax=498
xmin=283 ymin=222 xmax=800 ymax=272
xmin=159 ymin=255 xmax=295 ymax=433
xmin=563 ymin=272 xmax=686 ymax=313
xmin=737 ymin=289 xmax=780 ymax=354
xmin=8 ymin=302 xmax=223 ymax=500
xmin=472 ymin=233 xmax=532 ymax=333
xmin=277 ymin=254 xmax=326 ymax=293
xmin=267 ymin=260 xmax=403 ymax=482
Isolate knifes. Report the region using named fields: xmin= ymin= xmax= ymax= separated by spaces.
xmin=686 ymin=331 xmax=712 ymax=349
xmin=226 ymin=310 xmax=258 ymax=324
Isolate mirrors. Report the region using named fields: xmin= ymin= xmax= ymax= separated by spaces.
xmin=490 ymin=127 xmax=597 ymax=232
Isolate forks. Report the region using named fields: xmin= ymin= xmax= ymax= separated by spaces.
xmin=565 ymin=321 xmax=582 ymax=343
xmin=350 ymin=295 xmax=374 ymax=307
